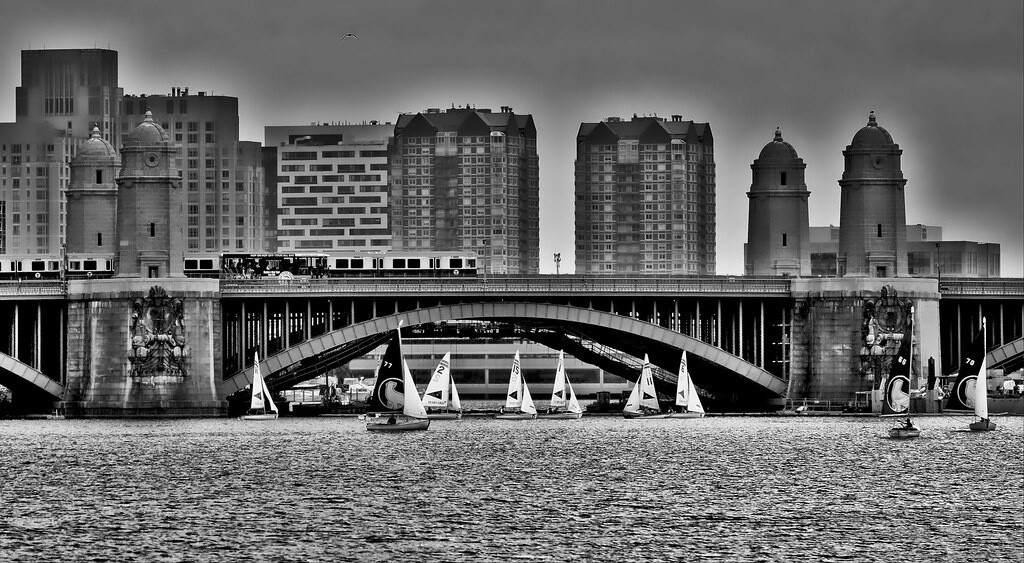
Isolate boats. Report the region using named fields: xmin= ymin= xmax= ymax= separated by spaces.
xmin=985 ymin=367 xmax=1023 ymax=414
xmin=776 ymin=397 xmax=846 ymax=416
xmin=840 ymin=389 xmax=886 ymax=417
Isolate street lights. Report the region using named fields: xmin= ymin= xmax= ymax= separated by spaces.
xmin=483 ymin=238 xmax=487 ymax=289
xmin=935 ymin=241 xmax=942 ymax=293
xmin=61 ymin=243 xmax=67 ymax=293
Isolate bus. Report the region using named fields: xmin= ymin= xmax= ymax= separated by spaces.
xmin=219 ymin=251 xmax=332 ymax=287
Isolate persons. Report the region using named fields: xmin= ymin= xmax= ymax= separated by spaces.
xmin=218 ymin=254 xmax=331 ymax=280
xmin=386 ymin=414 xmax=396 ymax=425
xmin=903 ymin=418 xmax=914 ymax=429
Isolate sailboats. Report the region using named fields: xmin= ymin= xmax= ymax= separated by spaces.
xmin=491 ymin=350 xmax=541 ymax=419
xmin=941 ymin=316 xmax=996 ymax=432
xmin=366 ymin=318 xmax=431 ymax=432
xmin=421 ymin=350 xmax=463 ymax=421
xmin=666 ymin=346 xmax=706 ymax=419
xmin=535 ymin=348 xmax=583 ymax=419
xmin=878 ymin=376 xmax=917 ymax=419
xmin=879 ymin=305 xmax=922 ymax=438
xmin=622 ymin=353 xmax=677 ymax=419
xmin=240 ymin=350 xmax=279 ymax=421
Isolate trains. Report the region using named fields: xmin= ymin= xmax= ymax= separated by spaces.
xmin=0 ymin=247 xmax=481 ymax=279
